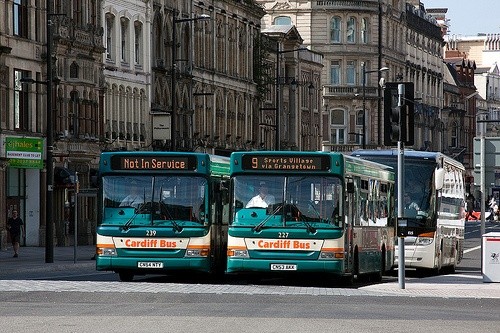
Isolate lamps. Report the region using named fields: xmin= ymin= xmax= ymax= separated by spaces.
xmin=104 ymin=131 xmax=146 ymax=147
xmin=260 ymin=143 xmax=264 ymax=146
xmin=237 ymin=135 xmax=241 ymax=140
xmin=205 ymin=134 xmax=211 ymax=138
xmin=216 ymin=135 xmax=219 ymax=140
xmin=194 ymin=132 xmax=200 ymax=138
xmin=252 ymin=140 xmax=257 ymax=144
xmin=246 ymin=140 xmax=251 ymax=143
xmin=226 ymin=133 xmax=232 ymax=139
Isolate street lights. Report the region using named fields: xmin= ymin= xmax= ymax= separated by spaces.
xmin=171 ymin=7 xmax=212 ymax=152
xmin=258 ymin=40 xmax=309 ymax=151
xmin=18 ymin=77 xmax=56 ymax=263
xmin=347 ymin=63 xmax=390 ymax=148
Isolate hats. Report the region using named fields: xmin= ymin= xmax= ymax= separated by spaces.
xmin=258 ymin=180 xmax=268 ymax=186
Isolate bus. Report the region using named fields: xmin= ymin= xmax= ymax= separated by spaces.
xmin=223 ymin=149 xmax=398 ymax=287
xmin=89 ymin=149 xmax=253 ymax=284
xmin=347 ymin=147 xmax=469 ymax=276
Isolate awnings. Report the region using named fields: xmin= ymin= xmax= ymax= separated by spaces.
xmin=55 ymin=167 xmax=80 ymax=187
xmin=90 ymin=168 xmax=99 ymax=188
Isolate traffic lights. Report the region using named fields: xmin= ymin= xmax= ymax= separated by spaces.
xmin=389 ymin=106 xmax=400 ymax=141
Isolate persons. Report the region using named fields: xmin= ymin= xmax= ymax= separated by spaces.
xmin=488 ymin=197 xmax=496 ymax=208
xmin=404 ymin=192 xmax=421 ymax=210
xmin=246 ymin=181 xmax=275 ymax=208
xmin=374 ymin=205 xmax=378 ymax=219
xmin=7 ymin=209 xmax=25 ymax=257
xmin=119 ymin=180 xmax=144 ymax=208
xmin=465 ymin=199 xmax=477 ymax=220
xmin=360 ymin=200 xmax=373 ymax=226
